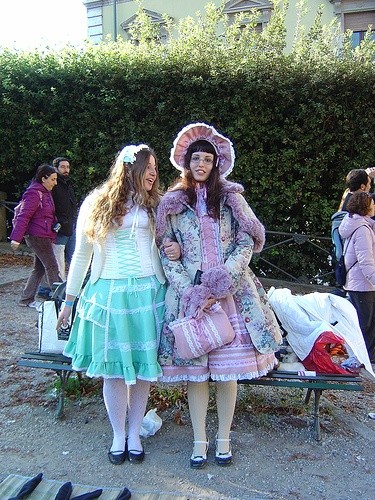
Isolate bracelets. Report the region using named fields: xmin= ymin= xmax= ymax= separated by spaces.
xmin=65 ymin=301 xmax=73 ymax=306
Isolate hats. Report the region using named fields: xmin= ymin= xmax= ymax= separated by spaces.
xmin=169 ymin=122 xmax=237 ymax=178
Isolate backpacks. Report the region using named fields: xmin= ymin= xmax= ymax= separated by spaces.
xmin=336 ymin=224 xmax=371 ymax=285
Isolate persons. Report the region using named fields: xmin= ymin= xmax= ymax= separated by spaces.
xmin=156 ymin=123 xmax=282 ymax=469
xmin=9 ymin=164 xmax=63 ymax=309
xmin=37 ymin=157 xmax=76 ymax=301
xmin=56 ymin=143 xmax=181 ymax=465
xmin=331 ymin=167 xmax=375 ymax=291
xmin=337 ymin=191 xmax=375 ymax=358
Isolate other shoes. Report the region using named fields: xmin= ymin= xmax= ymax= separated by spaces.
xmin=18 ymin=300 xmax=41 ymax=307
xmin=126 ymin=436 xmax=145 ymax=464
xmin=215 ymin=432 xmax=232 ymax=466
xmin=108 ymin=439 xmax=126 ymax=466
xmin=190 ymin=437 xmax=210 ymax=469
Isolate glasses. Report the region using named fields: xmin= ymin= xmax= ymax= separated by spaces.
xmin=191 ymin=156 xmax=213 ymax=165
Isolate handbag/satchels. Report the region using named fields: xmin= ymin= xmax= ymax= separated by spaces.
xmin=168 ymin=301 xmax=235 ymax=360
xmin=12 ymin=191 xmax=43 ymax=237
xmin=37 ymin=281 xmax=85 ymax=354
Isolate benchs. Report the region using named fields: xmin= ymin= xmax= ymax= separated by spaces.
xmin=17 ymin=350 xmax=365 ymax=443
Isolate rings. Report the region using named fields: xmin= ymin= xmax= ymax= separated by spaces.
xmin=170 ymin=254 xmax=174 ymax=257
